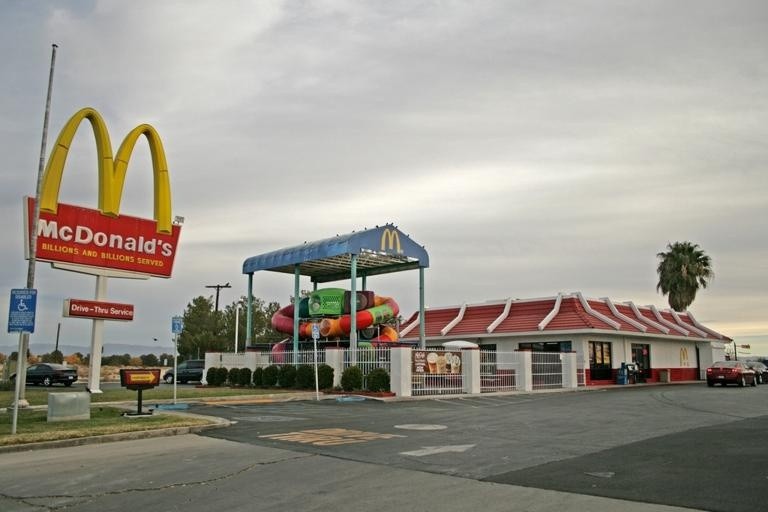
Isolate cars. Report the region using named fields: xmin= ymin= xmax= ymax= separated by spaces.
xmin=744 ymin=362 xmax=768 ymax=385
xmin=9 ymin=363 xmax=79 ymax=387
xmin=706 ymin=361 xmax=757 ymax=387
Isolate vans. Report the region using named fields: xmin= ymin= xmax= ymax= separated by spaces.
xmin=163 ymin=359 xmax=205 ymax=385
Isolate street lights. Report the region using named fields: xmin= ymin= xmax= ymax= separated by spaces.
xmin=234 ymin=302 xmax=243 ymax=354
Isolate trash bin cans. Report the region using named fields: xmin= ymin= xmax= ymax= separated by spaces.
xmin=660 ymin=368 xmax=671 ymax=383
